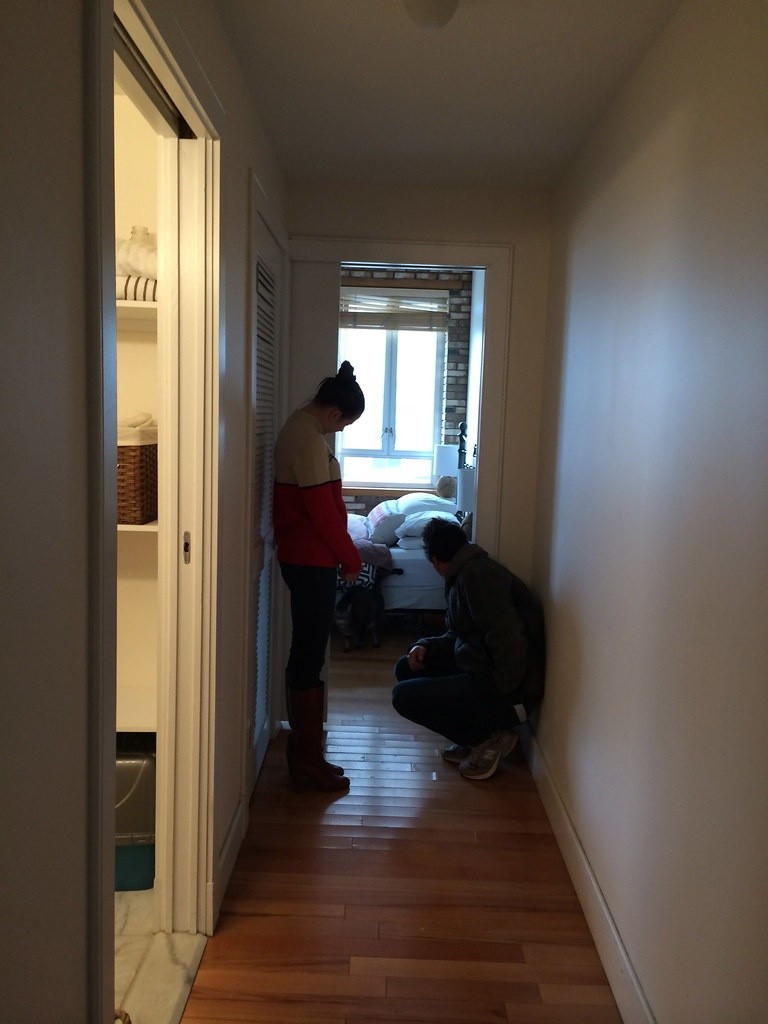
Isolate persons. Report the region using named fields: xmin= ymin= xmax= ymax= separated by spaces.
xmin=273 ymin=361 xmax=365 ymax=792
xmin=391 ymin=520 xmax=536 ymax=778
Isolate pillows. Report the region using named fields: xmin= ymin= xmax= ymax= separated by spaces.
xmin=363 ymin=492 xmax=460 ymax=550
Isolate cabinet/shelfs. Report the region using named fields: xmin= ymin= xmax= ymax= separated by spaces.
xmin=115 ymin=301 xmax=158 ymax=733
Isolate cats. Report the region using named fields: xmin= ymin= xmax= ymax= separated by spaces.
xmin=333 ymin=603 xmax=381 ymax=650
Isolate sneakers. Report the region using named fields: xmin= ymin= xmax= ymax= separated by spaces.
xmin=441 ymin=742 xmax=471 ymax=764
xmin=459 ymin=728 xmax=520 ymax=780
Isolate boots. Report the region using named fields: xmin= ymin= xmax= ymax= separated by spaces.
xmin=286 ymin=680 xmax=350 ymax=792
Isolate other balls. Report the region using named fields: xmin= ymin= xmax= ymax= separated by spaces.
xmin=436 ymin=475 xmax=457 ymax=498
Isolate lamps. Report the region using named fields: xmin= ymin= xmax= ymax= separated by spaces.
xmin=433 ymin=445 xmax=459 ymax=499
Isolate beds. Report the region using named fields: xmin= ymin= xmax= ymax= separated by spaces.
xmin=347 ymin=422 xmax=477 ymax=609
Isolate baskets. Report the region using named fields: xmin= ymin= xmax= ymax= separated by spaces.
xmin=117 ymin=428 xmax=158 ymax=525
